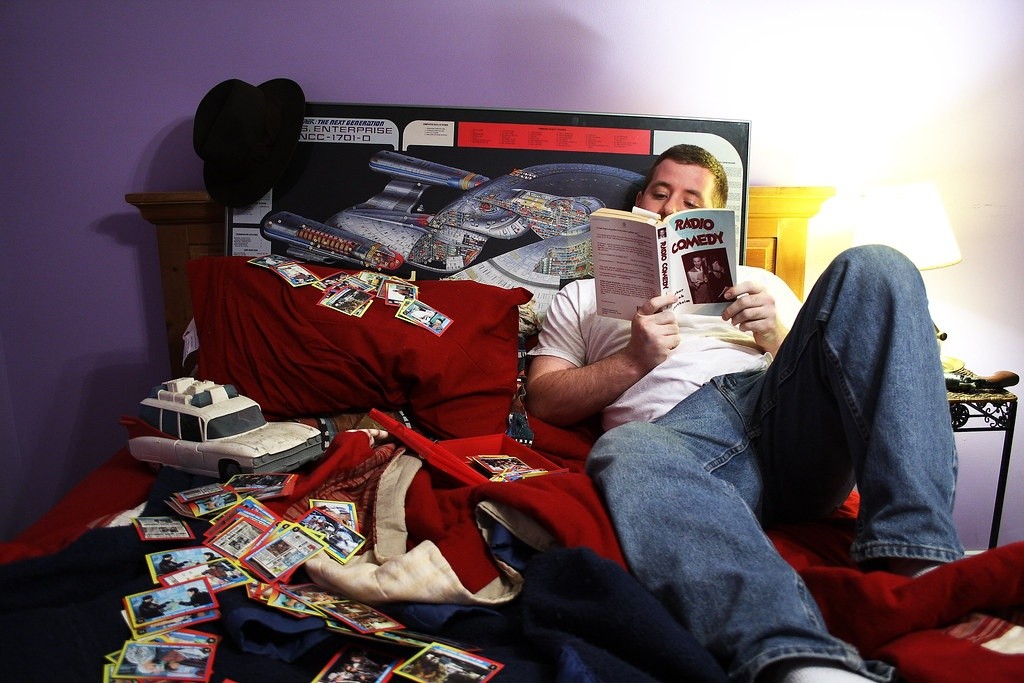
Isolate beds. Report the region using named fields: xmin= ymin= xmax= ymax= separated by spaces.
xmin=0 ymin=185 xmax=1024 ymax=683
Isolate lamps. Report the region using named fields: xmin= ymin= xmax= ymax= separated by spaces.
xmin=863 ymin=180 xmax=964 ymax=373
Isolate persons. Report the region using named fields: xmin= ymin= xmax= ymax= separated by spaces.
xmin=524 ymin=145 xmax=963 ymax=682
xmin=687 ymin=254 xmax=735 ymax=304
xmin=105 ymin=455 xmax=547 ymax=683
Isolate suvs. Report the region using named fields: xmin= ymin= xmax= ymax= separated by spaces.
xmin=128 ymin=376 xmax=327 ymax=484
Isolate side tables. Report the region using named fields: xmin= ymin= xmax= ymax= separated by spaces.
xmin=943 ymin=359 xmax=1018 ymax=551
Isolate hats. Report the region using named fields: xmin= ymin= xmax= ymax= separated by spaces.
xmin=192 ymin=77 xmax=306 ymax=209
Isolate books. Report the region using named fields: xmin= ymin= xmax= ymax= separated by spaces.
xmin=589 ymin=206 xmax=740 ymax=321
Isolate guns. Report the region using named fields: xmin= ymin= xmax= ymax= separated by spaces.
xmin=945 ymin=370 xmax=1021 ymax=391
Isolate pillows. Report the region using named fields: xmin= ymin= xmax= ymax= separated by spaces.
xmin=185 ymin=256 xmax=533 ymax=440
